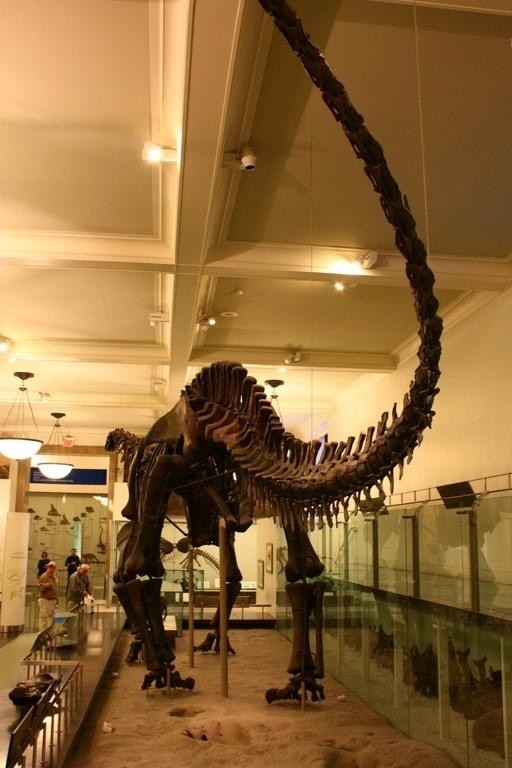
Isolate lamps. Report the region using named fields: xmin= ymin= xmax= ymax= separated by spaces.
xmin=1 ymin=370 xmax=75 ymax=484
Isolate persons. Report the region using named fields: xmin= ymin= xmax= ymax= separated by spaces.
xmin=66 ymin=564 xmax=92 ymax=611
xmin=65 ymin=549 xmax=80 ymax=598
xmin=38 ymin=551 xmax=51 ymax=578
xmin=39 ymin=561 xmax=59 ymax=634
